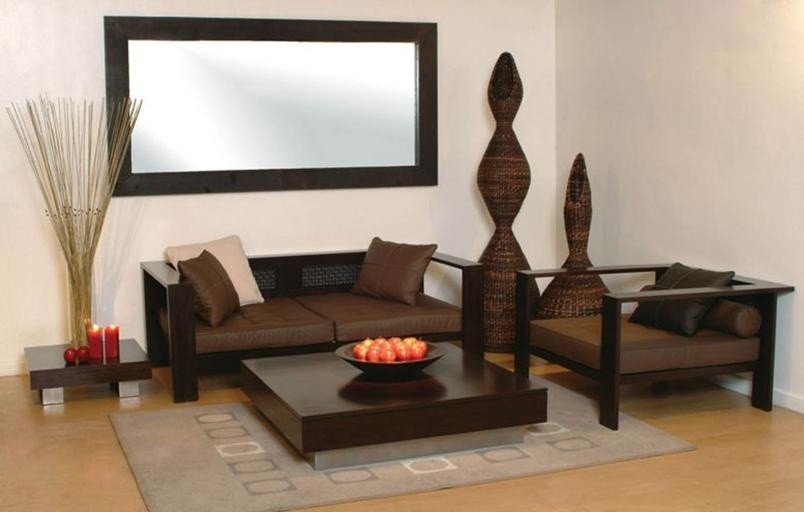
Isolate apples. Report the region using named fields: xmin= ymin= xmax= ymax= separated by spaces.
xmin=404 ymin=337 xmax=416 ymax=346
xmin=62 ymin=346 xmax=76 ymax=365
xmin=77 ymin=346 xmax=90 ymax=365
xmin=396 ymin=345 xmax=412 ymax=361
xmin=416 ymin=340 xmax=428 ymax=353
xmin=380 ymin=347 xmax=396 ymax=361
xmin=361 ymin=335 xmax=373 ymax=347
xmin=353 ymin=344 xmax=366 ymax=359
xmin=366 ymin=347 xmax=380 ymax=362
xmin=389 ymin=336 xmax=401 ymax=345
xmin=375 ymin=334 xmax=386 ymax=343
xmin=412 ymin=345 xmax=424 ymax=360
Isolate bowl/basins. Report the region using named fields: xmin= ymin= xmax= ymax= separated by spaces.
xmin=336 ymin=340 xmax=446 ymax=378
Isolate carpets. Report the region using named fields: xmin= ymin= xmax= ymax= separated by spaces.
xmin=109 ymin=370 xmax=696 ymax=512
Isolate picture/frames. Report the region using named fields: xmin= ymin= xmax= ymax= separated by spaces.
xmin=99 ymin=14 xmax=439 ymax=198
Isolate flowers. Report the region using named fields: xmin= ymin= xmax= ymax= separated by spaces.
xmin=3 ymin=92 xmax=143 ymax=346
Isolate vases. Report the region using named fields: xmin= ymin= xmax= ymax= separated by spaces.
xmin=66 ymin=262 xmax=97 ymax=353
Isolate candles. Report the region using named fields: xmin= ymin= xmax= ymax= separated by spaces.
xmin=88 ymin=329 xmax=103 ymax=363
xmin=105 ymin=326 xmax=119 ymax=363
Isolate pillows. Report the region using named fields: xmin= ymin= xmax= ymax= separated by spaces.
xmin=660 ymin=285 xmax=756 ymax=337
xmin=166 ymin=236 xmax=264 ymax=307
xmin=627 ymin=264 xmax=735 ymax=337
xmin=352 ymin=237 xmax=437 ymax=307
xmin=178 ymin=251 xmax=243 ymax=327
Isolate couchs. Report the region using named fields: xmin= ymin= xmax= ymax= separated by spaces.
xmin=517 ymin=264 xmax=795 ymax=429
xmin=142 ymin=249 xmax=484 ymax=404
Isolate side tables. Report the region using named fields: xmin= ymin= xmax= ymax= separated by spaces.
xmin=24 ymin=338 xmax=152 ymax=405
